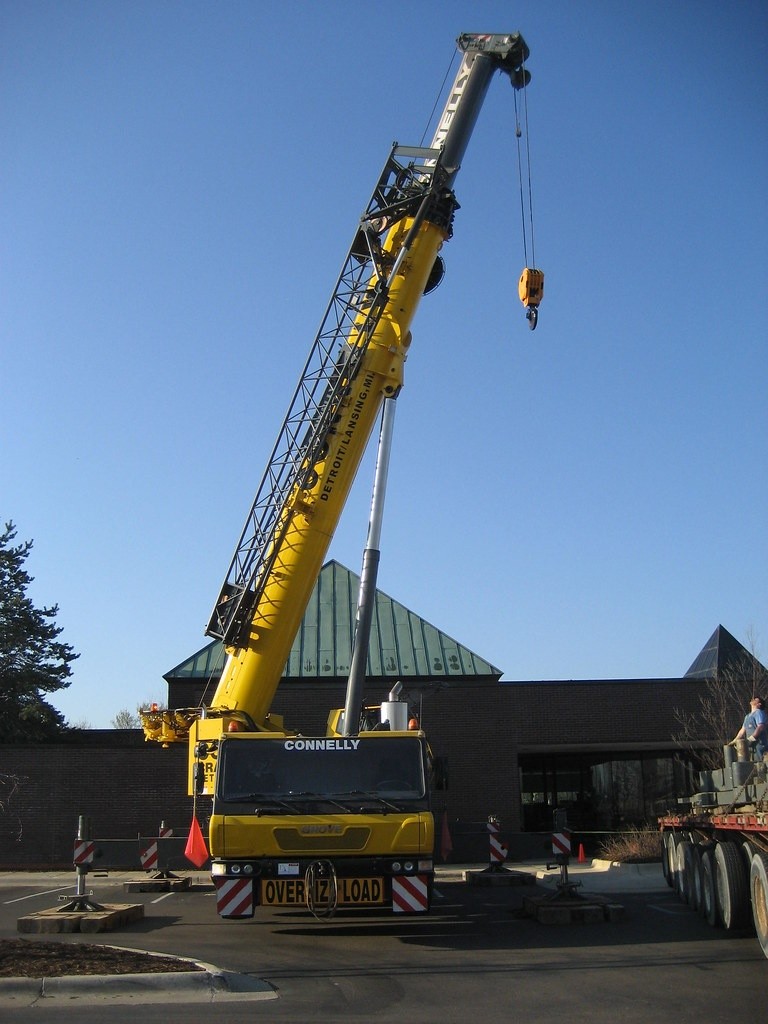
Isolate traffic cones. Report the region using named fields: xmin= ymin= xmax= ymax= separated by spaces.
xmin=576 ymin=843 xmax=589 ymax=863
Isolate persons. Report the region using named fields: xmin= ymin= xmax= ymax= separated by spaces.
xmin=728 ymin=697 xmax=768 ymax=762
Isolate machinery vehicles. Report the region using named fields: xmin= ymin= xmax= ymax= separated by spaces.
xmin=56 ymin=30 xmax=590 ymax=924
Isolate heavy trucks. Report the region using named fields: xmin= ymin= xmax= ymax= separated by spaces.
xmin=654 ymin=813 xmax=768 ymax=963
